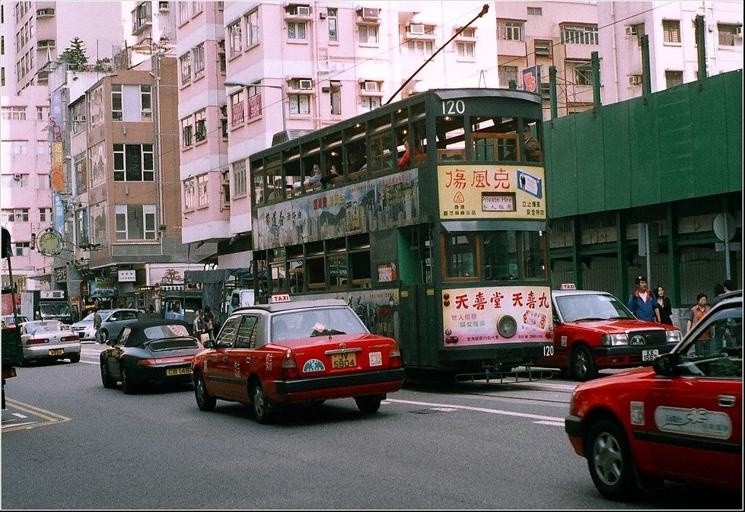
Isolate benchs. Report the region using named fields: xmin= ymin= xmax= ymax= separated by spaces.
xmin=309 ymin=283 xmax=325 ymax=290
xmin=351 ymin=278 xmax=371 ymax=288
xmin=286 ymin=149 xmax=466 ymax=198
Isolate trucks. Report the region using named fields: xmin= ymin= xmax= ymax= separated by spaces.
xmin=3 ymin=227 xmax=25 ymax=409
xmin=20 ymin=289 xmax=74 ymax=326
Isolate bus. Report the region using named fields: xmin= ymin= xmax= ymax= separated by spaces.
xmin=247 ymin=4 xmax=555 ymax=392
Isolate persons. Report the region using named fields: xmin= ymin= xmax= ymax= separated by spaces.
xmin=203 ymin=305 xmax=215 ymax=343
xmin=320 ymin=162 xmax=344 ymax=185
xmin=627 ymin=275 xmax=662 ymax=324
xmin=398 ymin=134 xmax=424 ymax=167
xmin=653 ymin=286 xmax=674 ymax=326
xmin=268 ymin=177 xmax=285 ymax=201
xmin=505 ymin=124 xmax=542 ymax=162
xmin=192 ymin=304 xmax=205 ymax=335
xmin=686 ymin=294 xmax=715 ymax=355
xmin=292 ymin=162 xmax=321 ymax=187
xmin=147 ymin=305 xmax=153 ymax=314
xmin=93 ymin=309 xmax=102 ymax=346
xmin=714 ymin=278 xmax=738 ymax=349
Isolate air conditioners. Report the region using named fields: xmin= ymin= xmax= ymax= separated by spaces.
xmin=297 ymin=6 xmax=309 ymax=16
xmin=409 ymin=25 xmax=424 ymax=35
xmin=361 ymin=8 xmax=380 ymax=22
xmin=629 ymin=76 xmax=642 ymax=85
xmin=625 ymin=27 xmax=638 ymax=35
xmin=365 ymin=81 xmax=379 ymax=92
xmin=300 ymin=80 xmax=312 ymax=90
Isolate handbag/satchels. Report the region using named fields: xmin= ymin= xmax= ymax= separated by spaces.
xmin=206 ymin=320 xmax=214 ymax=333
xmin=201 ymin=333 xmax=209 ymax=344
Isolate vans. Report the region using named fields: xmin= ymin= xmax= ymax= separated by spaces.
xmin=3 ymin=312 xmax=29 ymax=334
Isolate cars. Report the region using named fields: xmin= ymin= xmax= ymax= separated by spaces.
xmin=533 ymin=282 xmax=683 ymax=383
xmin=563 ymin=290 xmax=740 ymax=506
xmin=72 ymin=307 xmax=146 ymax=342
xmin=100 ymin=317 xmax=205 ymax=395
xmin=191 ymin=292 xmax=405 ymax=424
xmin=7 ymin=318 xmax=82 ymax=368
